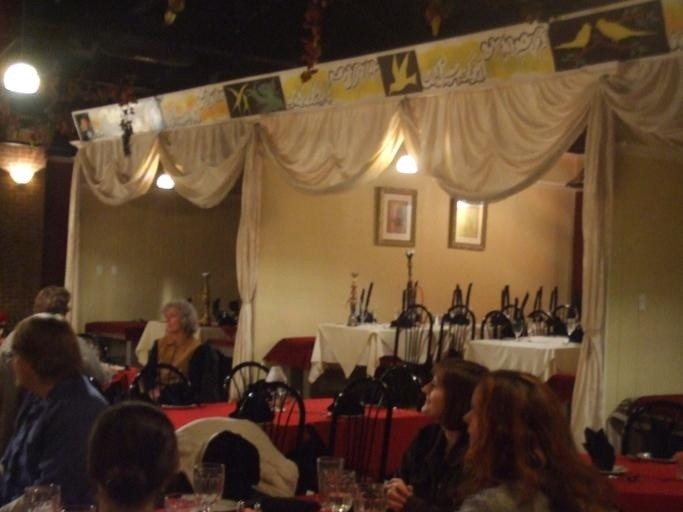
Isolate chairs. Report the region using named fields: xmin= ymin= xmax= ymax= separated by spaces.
xmin=479 ymin=310 xmax=514 ymax=340
xmin=128 ymin=362 xmax=188 ymax=405
xmin=238 ymin=380 xmax=305 ymax=450
xmin=328 ymin=378 xmax=393 ymax=481
xmin=527 ymin=310 xmax=553 ymax=333
xmin=553 ymin=304 xmax=571 ymax=322
xmin=394 ymin=304 xmax=433 ymax=363
xmin=502 ymin=304 xmax=524 ymax=335
xmin=223 ymin=361 xmax=272 ymax=401
xmin=435 ymin=305 xmax=475 ymax=363
xmin=620 ymin=398 xmax=683 ymax=459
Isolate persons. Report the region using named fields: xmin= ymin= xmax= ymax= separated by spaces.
xmin=138 ymin=300 xmax=204 ymax=403
xmin=0 ymin=286 xmax=113 ymax=398
xmin=86 ymin=401 xmax=180 ymax=512
xmin=460 ymin=368 xmax=615 ymax=512
xmin=0 ymin=312 xmax=110 ymax=512
xmin=382 ymin=355 xmax=491 ymax=512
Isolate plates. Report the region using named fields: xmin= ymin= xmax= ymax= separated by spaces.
xmin=603 ymin=464 xmax=628 ymax=475
xmin=644 ymin=452 xmax=677 ymax=463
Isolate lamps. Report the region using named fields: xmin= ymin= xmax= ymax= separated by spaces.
xmin=0 ymin=1 xmax=47 ymax=101
xmin=0 ymin=118 xmax=47 ymax=187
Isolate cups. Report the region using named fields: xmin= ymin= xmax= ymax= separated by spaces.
xmin=192 ymin=463 xmax=224 ymax=511
xmin=354 ymin=483 xmax=384 ymax=512
xmin=24 ymin=485 xmax=60 ymax=511
xmin=319 ymin=469 xmax=355 ymax=512
xmin=317 ymin=456 xmax=344 ymax=484
xmin=165 ymin=494 xmax=194 ymax=511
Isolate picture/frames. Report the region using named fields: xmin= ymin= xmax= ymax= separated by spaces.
xmin=376 ymin=186 xmax=417 ymax=248
xmin=449 ymin=196 xmax=488 ymax=251
